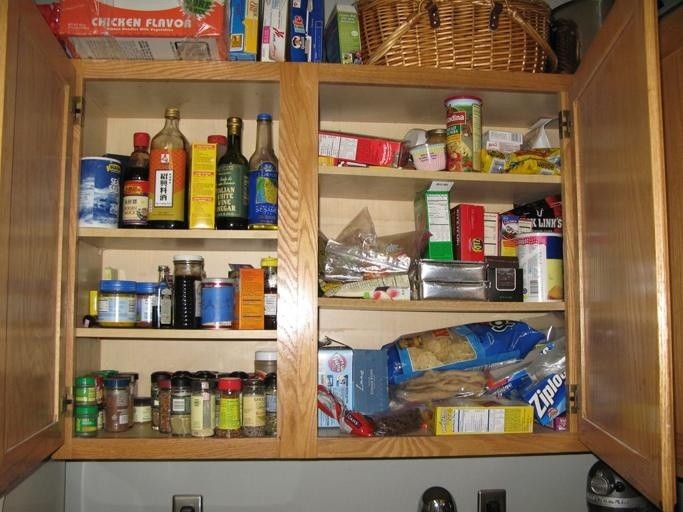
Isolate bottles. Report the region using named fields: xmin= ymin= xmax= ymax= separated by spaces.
xmin=170 ymin=376 xmax=191 ymax=438
xmin=215 ymin=117 xmax=249 ymax=229
xmin=249 ymin=112 xmax=279 ymax=229
xmin=265 ymin=372 xmax=277 ymax=436
xmin=445 ymin=96 xmax=483 ymax=171
xmin=152 ymin=267 xmax=172 ymax=328
xmin=74 ymin=377 xmax=96 ymax=405
xmin=254 ymin=352 xmax=276 ymax=376
xmin=159 ymin=381 xmax=171 ymax=433
xmin=97 ymin=280 xmax=138 ymax=327
xmin=199 ymin=278 xmax=234 ymax=328
xmin=120 ymin=133 xmax=149 ymax=228
xmin=208 ymin=135 xmax=227 ymax=159
xmin=78 ymin=156 xmax=120 ymax=228
xmin=120 ymin=372 xmax=139 ymax=397
xmin=75 ymin=408 xmax=98 ymax=436
xmin=191 ymin=378 xmax=215 ymax=436
xmin=261 ymin=259 xmax=277 ymax=329
xmin=216 ymin=377 xmax=242 ymax=437
xmin=133 ymin=397 xmax=152 ymax=423
xmin=152 ymin=371 xmax=168 ymax=426
xmin=147 ymin=108 xmax=190 ymax=229
xmin=174 ymin=369 xmax=260 ymax=379
xmin=426 ymin=129 xmax=447 ymax=145
xmin=136 ymin=283 xmax=159 ymax=328
xmin=104 ymin=378 xmax=130 ymax=434
xmin=87 ymin=375 xmax=105 ymax=404
xmin=96 ymin=405 xmax=105 ymax=430
xmin=241 ymin=378 xmax=265 ymax=437
xmin=174 ymin=255 xmax=206 ymax=328
xmin=93 ymin=369 xmax=115 ymax=378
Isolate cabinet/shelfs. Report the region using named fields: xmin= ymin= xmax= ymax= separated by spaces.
xmin=0 ymin=0 xmax=296 ymax=495
xmin=296 ymin=0 xmax=683 ymax=510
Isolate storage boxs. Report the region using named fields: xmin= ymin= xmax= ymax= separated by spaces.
xmin=59 ymin=0 xmax=226 ymax=60
xmin=318 ymin=336 xmax=388 ymax=428
